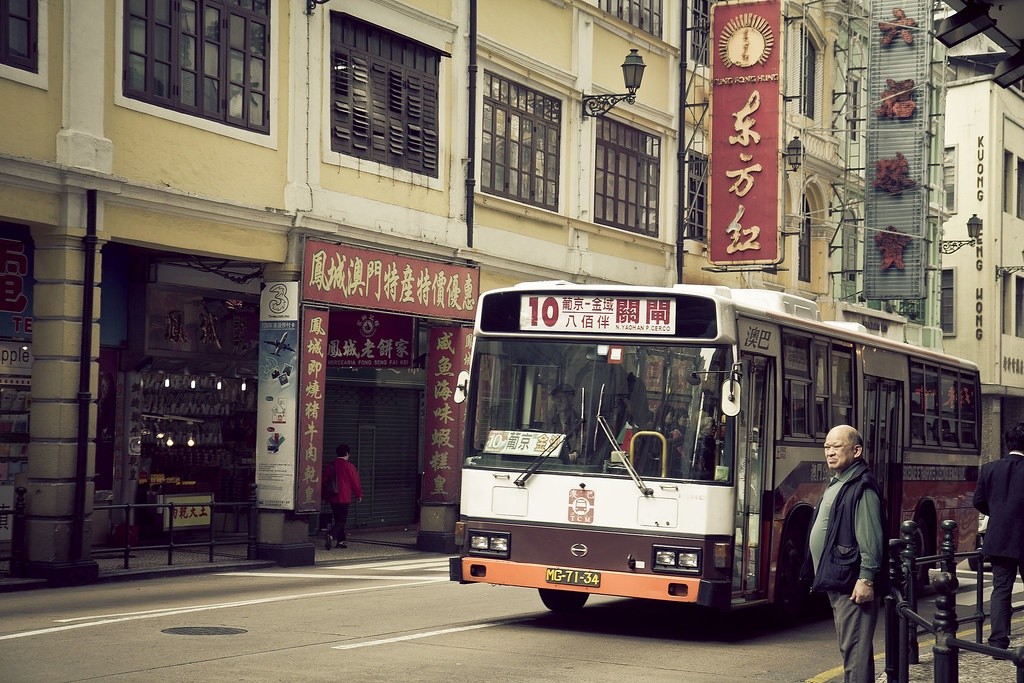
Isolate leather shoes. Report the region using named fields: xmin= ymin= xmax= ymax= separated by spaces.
xmin=336 ymin=542 xmax=347 ymax=549
xmin=325 ymin=533 xmax=332 ymax=551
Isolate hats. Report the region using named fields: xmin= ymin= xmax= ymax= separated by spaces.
xmin=550 ymin=383 xmax=575 ymax=394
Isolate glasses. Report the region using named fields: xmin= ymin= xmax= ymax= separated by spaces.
xmin=551 ymin=395 xmax=570 ymax=402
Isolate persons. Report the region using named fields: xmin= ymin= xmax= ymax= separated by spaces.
xmin=696 ymin=417 xmax=716 ymax=471
xmin=672 ymin=407 xmax=688 ymax=454
xmin=539 ymin=385 xmax=585 ymax=462
xmin=799 ymin=426 xmax=888 ymax=681
xmin=656 ymin=404 xmax=674 ymax=438
xmin=973 ymin=423 xmax=1024 ymax=660
xmin=321 ymin=446 xmax=361 ymax=549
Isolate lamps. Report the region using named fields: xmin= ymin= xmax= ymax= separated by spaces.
xmin=783 ymin=136 xmax=806 ymax=173
xmin=939 ymin=214 xmax=983 ymax=254
xmin=580 ymin=48 xmax=647 ymax=120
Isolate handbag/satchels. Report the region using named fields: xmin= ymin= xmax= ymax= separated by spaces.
xmin=323 ymin=460 xmax=339 ymax=501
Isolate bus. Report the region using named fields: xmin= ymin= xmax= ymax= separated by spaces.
xmin=444 ymin=277 xmax=984 ymax=627
